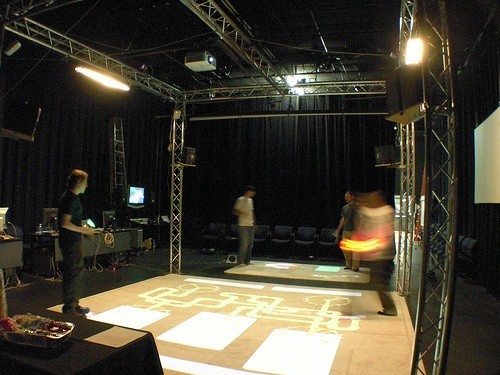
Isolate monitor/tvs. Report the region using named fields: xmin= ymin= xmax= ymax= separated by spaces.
xmin=127 ymin=184 xmax=145 ymax=209
xmin=41 ymin=208 xmax=58 ymax=232
xmin=0 ymin=207 xmax=9 ymax=235
xmin=102 ymin=211 xmax=116 ymax=232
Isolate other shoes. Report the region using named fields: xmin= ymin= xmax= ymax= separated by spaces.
xmin=344 ymin=267 xmax=352 ymax=269
xmin=377 ymin=311 xmax=397 ymax=316
xmin=75 ymin=306 xmax=90 ymax=313
xmin=63 ymin=310 xmax=86 ymax=319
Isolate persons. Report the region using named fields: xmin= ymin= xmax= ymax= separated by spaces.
xmin=339 ymin=191 xmax=398 ymax=316
xmin=57 ymin=170 xmax=95 ymax=320
xmin=332 ymin=191 xmax=364 ymax=272
xmin=232 ymin=186 xmax=257 ymax=267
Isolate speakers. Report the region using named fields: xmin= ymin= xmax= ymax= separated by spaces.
xmin=386 ymin=63 xmax=429 ymax=125
xmin=181 ymin=146 xmax=197 ymax=165
xmin=172 ymin=109 xmax=181 ymax=119
xmin=374 ymin=145 xmax=396 ymax=164
xmin=3 ymin=40 xmax=21 ymax=56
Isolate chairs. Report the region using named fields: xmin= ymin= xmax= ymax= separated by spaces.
xmin=201 ymin=223 xmax=342 ymax=261
xmin=431 ymin=230 xmax=478 ymax=280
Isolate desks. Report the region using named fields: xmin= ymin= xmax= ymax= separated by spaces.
xmin=0 ymin=304 xmax=165 ymax=375
xmin=0 ymin=230 xmax=134 ymax=288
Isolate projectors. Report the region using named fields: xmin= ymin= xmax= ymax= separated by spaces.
xmin=185 ymin=51 xmax=217 ymax=71
